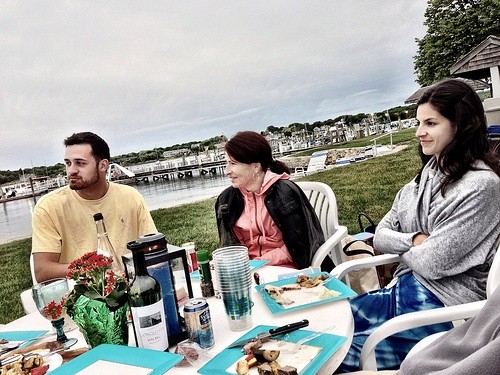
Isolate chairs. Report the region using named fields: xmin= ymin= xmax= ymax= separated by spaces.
xmin=20 ymin=243 xmax=180 ymax=315
xmin=294 ymin=181 xmax=500 ymax=375
xmin=290 ymin=149 xmax=328 ymax=179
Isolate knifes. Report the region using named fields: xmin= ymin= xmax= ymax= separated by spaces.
xmin=228 ymin=320 xmax=309 ymax=349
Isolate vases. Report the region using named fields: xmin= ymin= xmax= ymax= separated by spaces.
xmin=51 ymin=318 xmax=68 ymax=343
xmin=67 ymin=290 xmax=129 ymax=350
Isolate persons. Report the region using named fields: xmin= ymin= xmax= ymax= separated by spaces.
xmin=215 ymin=131 xmax=335 ymax=275
xmin=334 ymin=80 xmax=500 ymax=375
xmin=31 ymin=132 xmax=159 ymax=286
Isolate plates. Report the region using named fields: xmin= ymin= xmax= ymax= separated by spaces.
xmin=44 ymin=344 xmax=184 ymax=375
xmin=182 ymin=260 xmax=270 ymax=280
xmin=0 ymin=349 xmax=62 ymax=375
xmin=196 ymin=324 xmax=348 ymax=374
xmin=0 ymin=329 xmax=49 ymax=359
xmin=255 ymin=271 xmax=359 ymax=316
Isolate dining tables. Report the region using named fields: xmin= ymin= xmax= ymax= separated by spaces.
xmin=0 ymin=260 xmax=354 ymax=375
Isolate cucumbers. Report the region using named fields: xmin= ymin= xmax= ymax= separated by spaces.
xmin=252 ymin=349 xmax=280 ymax=362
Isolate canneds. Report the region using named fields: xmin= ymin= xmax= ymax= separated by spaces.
xmin=182 ymin=242 xmax=198 ymax=273
xmin=183 ymin=298 xmax=215 ymax=352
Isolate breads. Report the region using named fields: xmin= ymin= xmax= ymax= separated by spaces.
xmin=264 ymin=285 xmax=284 ymax=294
xmin=282 ymin=283 xmax=301 ymax=290
xmin=173 ymin=346 xmax=199 ymax=366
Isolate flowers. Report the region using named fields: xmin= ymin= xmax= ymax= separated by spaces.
xmin=44 ymin=297 xmax=64 ymax=320
xmin=66 ymin=251 xmax=130 ymax=311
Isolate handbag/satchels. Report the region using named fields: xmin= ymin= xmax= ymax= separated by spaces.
xmin=341 ymin=213 xmax=399 ymax=296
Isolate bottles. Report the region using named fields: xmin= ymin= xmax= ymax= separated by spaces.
xmin=126 ymin=243 xmax=170 ymax=353
xmin=180 ymin=241 xmax=198 ymax=273
xmin=208 ymin=260 xmax=222 ymax=298
xmin=196 ymin=250 xmax=213 ymax=297
xmin=93 ymin=213 xmax=124 ymax=277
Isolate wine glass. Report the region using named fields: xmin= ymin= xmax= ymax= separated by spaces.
xmin=32 ymin=278 xmax=78 ymax=350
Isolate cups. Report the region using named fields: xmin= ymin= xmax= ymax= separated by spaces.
xmin=212 ymin=246 xmax=254 ymax=332
